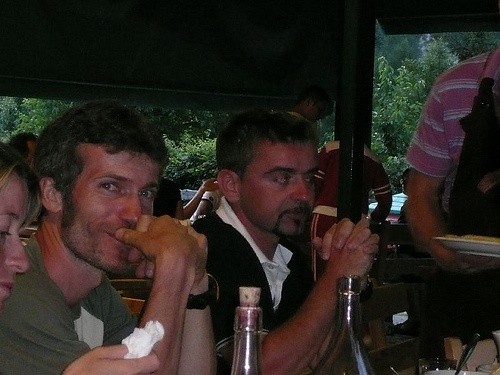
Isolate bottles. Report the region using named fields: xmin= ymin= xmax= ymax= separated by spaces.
xmin=230 ymin=285 xmax=262 ymax=375
xmin=312 ymin=274 xmax=374 ymax=375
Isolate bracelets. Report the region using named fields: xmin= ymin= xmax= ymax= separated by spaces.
xmin=187 ymin=291 xmax=212 ymax=312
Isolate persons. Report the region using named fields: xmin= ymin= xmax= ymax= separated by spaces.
xmin=154 ymin=173 xmax=220 ymax=225
xmin=404 ymin=48 xmax=500 ymax=375
xmin=191 ymin=109 xmax=380 ymax=375
xmin=0 ymin=100 xmax=216 ymax=375
xmin=315 ymin=136 xmax=392 ymax=224
xmin=291 ymin=84 xmax=333 ymax=123
xmin=0 ymin=143 xmax=161 ymax=375
xmin=8 ymin=131 xmax=40 ymax=169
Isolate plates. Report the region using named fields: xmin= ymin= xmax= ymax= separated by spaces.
xmin=431 ymin=236 xmax=500 ymax=258
xmin=425 ymin=369 xmax=489 ymax=374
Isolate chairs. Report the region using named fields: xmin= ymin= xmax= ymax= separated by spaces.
xmin=370 ymin=222 xmax=433 ymax=336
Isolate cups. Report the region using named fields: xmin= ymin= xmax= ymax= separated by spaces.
xmin=419 ymin=357 xmax=456 ymax=375
xmin=493 ymin=330 xmax=500 ymax=352
